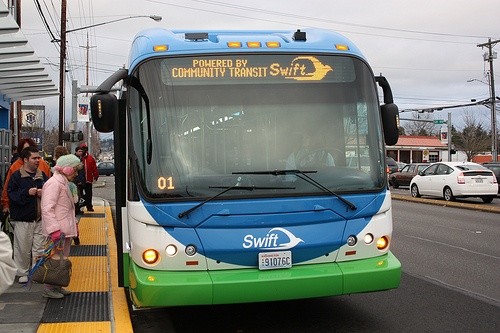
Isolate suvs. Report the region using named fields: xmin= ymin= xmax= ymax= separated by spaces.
xmin=346 ymin=153 xmax=399 ymax=187
xmin=477 ymin=162 xmax=500 ymax=194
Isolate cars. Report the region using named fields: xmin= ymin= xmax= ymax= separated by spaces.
xmin=389 ymin=162 xmax=431 ymax=189
xmin=409 ymin=162 xmax=499 ymax=203
xmin=96 ymin=151 xmax=115 ymax=176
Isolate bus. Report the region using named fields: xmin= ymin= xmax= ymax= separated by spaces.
xmin=87 ymin=25 xmax=404 ymax=318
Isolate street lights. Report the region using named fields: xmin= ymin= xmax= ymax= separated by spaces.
xmin=57 ymin=14 xmax=163 ymax=147
xmin=466 ymin=78 xmax=498 ymax=162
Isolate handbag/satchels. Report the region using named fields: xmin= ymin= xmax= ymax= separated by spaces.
xmin=2 ymin=212 xmax=16 ymax=260
xmin=31 ymin=234 xmax=73 ymax=288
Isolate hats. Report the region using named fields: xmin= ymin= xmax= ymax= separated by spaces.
xmin=80 ymin=143 xmax=86 ymax=148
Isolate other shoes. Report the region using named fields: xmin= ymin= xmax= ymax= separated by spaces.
xmin=76 ymin=210 xmax=84 ymax=215
xmin=87 ymin=206 xmax=94 ymax=211
xmin=18 ymin=276 xmax=28 ymax=283
xmin=42 ymin=286 xmax=71 ymax=298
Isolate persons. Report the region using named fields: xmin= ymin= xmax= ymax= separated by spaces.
xmin=50 ymin=143 xmax=99 ymax=215
xmin=40 ymin=154 xmax=82 ymax=298
xmin=2 ymin=138 xmax=52 ymax=284
xmin=285 ymin=129 xmax=335 ymax=182
